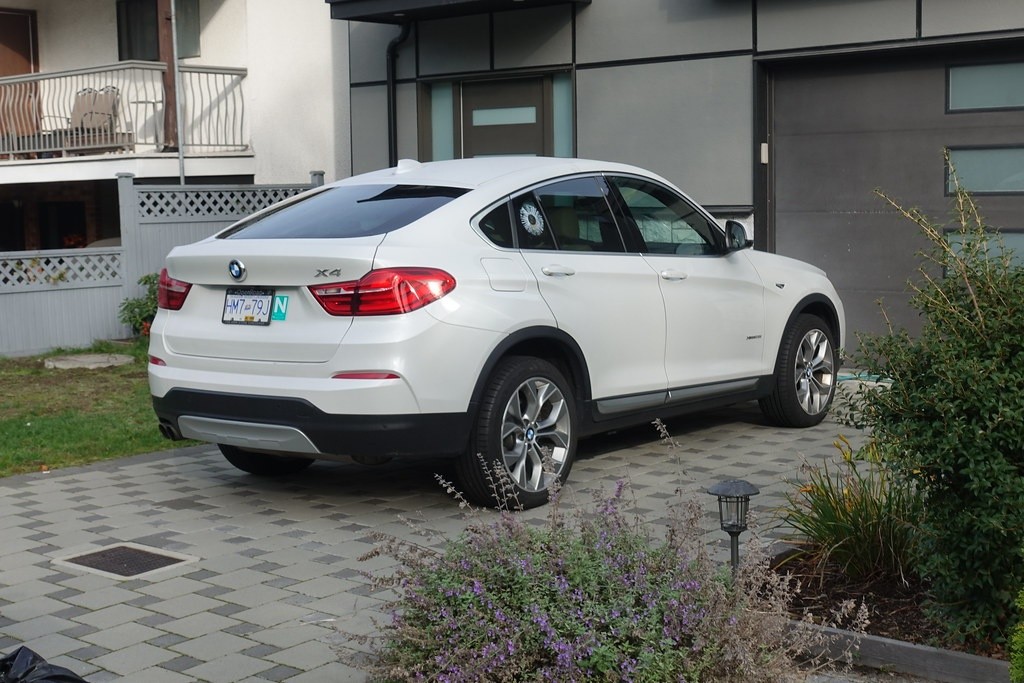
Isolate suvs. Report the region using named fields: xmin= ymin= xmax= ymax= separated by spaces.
xmin=146 ymin=154 xmax=848 ymax=512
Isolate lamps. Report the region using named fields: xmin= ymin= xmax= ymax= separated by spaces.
xmin=704 ymin=478 xmax=760 ymax=577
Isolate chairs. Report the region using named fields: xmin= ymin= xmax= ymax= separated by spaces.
xmin=528 ymin=206 xmax=594 ymax=251
xmin=34 ymin=85 xmax=119 ymax=158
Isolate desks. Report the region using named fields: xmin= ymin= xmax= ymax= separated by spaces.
xmin=129 ymin=99 xmax=166 ymax=152
